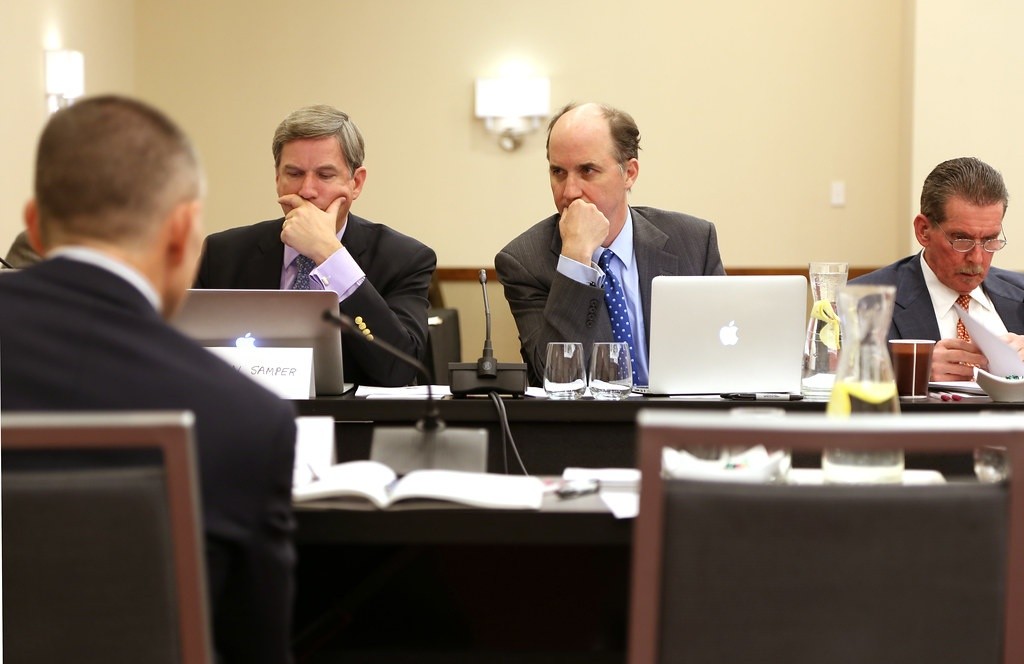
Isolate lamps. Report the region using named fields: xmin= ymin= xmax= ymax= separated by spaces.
xmin=474 ymin=71 xmax=550 ymax=151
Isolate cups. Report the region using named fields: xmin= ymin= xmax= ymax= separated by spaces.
xmin=543 ymin=342 xmax=587 ymax=397
xmin=589 ymin=342 xmax=633 ymax=399
xmin=889 ymin=338 xmax=935 ymax=397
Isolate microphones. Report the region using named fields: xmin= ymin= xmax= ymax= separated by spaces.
xmin=447 ymin=269 xmax=527 ymax=397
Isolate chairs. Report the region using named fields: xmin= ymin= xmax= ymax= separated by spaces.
xmin=1 ymin=407 xmax=214 ymax=664
xmin=628 ymin=406 xmax=1024 ymax=664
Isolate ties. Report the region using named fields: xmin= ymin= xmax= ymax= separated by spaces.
xmin=597 ymin=249 xmax=639 ymax=386
xmin=955 ymin=294 xmax=972 ymax=382
xmin=290 ymin=255 xmax=316 ymax=290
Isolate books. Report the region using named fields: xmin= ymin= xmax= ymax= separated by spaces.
xmin=294 ymin=461 xmax=543 ymax=512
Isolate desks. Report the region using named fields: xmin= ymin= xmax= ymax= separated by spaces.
xmin=263 ymin=381 xmax=1024 ymax=548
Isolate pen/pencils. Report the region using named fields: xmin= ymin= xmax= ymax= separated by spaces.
xmin=928 ymin=390 xmax=962 ymax=401
xmin=721 ymin=393 xmax=804 ymax=400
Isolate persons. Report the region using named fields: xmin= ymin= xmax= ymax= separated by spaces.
xmin=495 ymin=102 xmax=729 ymax=653
xmin=0 ymin=95 xmax=296 ymax=664
xmin=847 ymin=158 xmax=1024 ymax=382
xmin=190 ymin=105 xmax=438 ymax=388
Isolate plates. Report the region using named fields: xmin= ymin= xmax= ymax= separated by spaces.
xmin=974 ymin=367 xmax=1024 ymax=403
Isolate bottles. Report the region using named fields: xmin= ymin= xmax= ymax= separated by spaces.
xmin=820 ymin=284 xmax=905 ymax=469
xmin=803 ymin=262 xmax=850 ymax=394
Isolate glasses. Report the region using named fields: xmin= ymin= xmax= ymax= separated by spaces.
xmin=934 ymin=220 xmax=1007 ymax=252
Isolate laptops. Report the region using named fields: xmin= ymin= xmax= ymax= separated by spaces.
xmin=164 ymin=288 xmax=355 ymax=394
xmin=633 ymin=276 xmax=808 ymax=396
